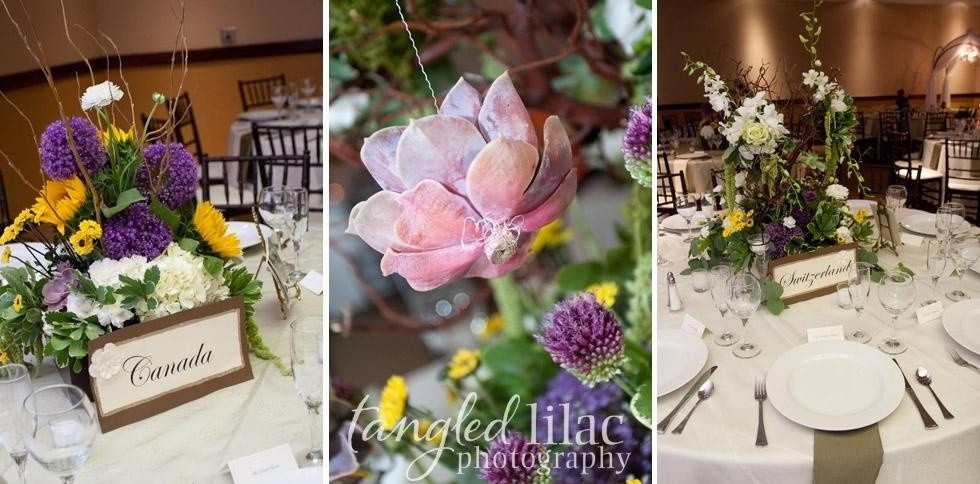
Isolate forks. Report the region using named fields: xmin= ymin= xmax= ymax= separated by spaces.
xmin=754 ymin=373 xmax=769 ymax=447
xmin=949 ymin=350 xmax=980 ymax=375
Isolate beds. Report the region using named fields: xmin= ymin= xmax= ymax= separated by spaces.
xmin=0 ymin=212 xmax=323 ymax=484
xmin=657 ymin=200 xmax=980 ymax=484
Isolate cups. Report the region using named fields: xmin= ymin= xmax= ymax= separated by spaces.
xmin=0 ymin=364 xmax=98 ymax=484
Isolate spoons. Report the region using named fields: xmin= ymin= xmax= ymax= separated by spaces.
xmin=670 ymin=380 xmax=714 ymax=434
xmin=915 ymin=366 xmax=954 ymax=420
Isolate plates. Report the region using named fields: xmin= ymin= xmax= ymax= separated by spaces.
xmin=767 ymin=337 xmax=906 ymax=433
xmin=236 ymin=110 xmax=287 ymax=121
xmin=658 ymin=329 xmax=708 ymax=399
xmin=2 ymin=240 xmax=66 ymax=290
xmin=226 ymin=221 xmax=272 ymax=250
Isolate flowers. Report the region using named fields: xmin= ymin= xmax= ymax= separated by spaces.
xmin=680 ymin=13 xmax=915 ymax=315
xmin=0 ymin=81 xmax=294 ymax=376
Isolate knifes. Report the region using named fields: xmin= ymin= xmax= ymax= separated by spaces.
xmin=892 ymin=358 xmax=938 ymax=431
xmin=656 ymin=366 xmax=718 ymax=435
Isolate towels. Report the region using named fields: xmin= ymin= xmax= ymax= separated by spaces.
xmin=812 ymin=422 xmax=884 ymax=484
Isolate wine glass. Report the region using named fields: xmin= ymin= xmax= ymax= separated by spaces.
xmin=926 ymin=117 xmax=980 ymax=147
xmin=657 ymin=183 xmax=979 ymax=359
xmin=290 ymin=315 xmax=322 ymax=469
xmin=259 ymin=186 xmax=310 ymax=283
xmin=270 ymin=77 xmax=323 ymax=121
xmin=657 ymin=129 xmax=722 ymax=159
